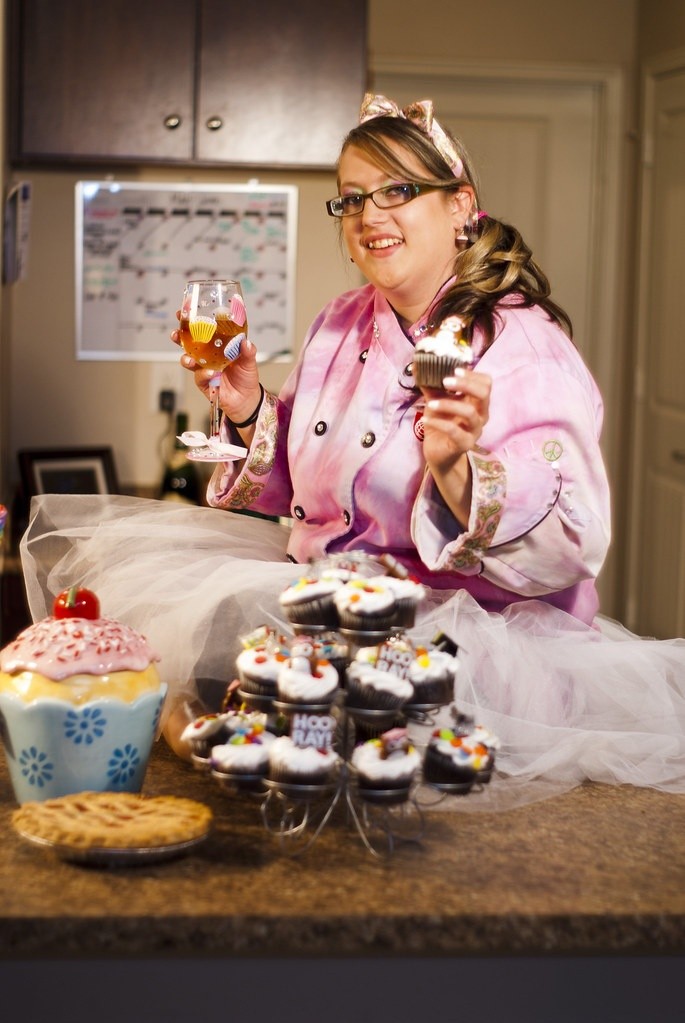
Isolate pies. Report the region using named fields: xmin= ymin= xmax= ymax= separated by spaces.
xmin=12 ymin=791 xmax=211 ymax=848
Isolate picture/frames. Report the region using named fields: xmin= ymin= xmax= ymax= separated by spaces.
xmin=17 ymin=445 xmax=125 ymax=500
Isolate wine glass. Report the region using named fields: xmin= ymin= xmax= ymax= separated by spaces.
xmin=181 ymin=279 xmax=247 ymax=463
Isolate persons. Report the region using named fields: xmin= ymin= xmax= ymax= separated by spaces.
xmin=170 ymin=92 xmax=613 ymax=771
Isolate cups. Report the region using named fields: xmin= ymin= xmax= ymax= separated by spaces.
xmin=0 ymin=582 xmax=168 ymax=808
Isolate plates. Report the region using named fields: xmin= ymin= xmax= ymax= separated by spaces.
xmin=17 ymin=831 xmax=210 ymax=857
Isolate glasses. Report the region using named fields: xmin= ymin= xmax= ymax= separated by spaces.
xmin=324 ymin=183 xmax=457 ymax=218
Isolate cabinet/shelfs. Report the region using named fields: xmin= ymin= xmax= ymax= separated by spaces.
xmin=8 ymin=0 xmax=368 ymax=174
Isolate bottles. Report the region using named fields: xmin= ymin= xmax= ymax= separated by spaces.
xmin=160 ymin=411 xmax=202 ymax=507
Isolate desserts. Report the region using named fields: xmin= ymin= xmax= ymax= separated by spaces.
xmin=180 ymin=551 xmax=502 ymax=807
xmin=1 ymin=587 xmax=166 ymax=810
xmin=414 ymin=317 xmax=475 ymax=391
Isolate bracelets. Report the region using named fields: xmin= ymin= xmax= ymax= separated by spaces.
xmin=226 ymin=382 xmax=264 ymax=428
